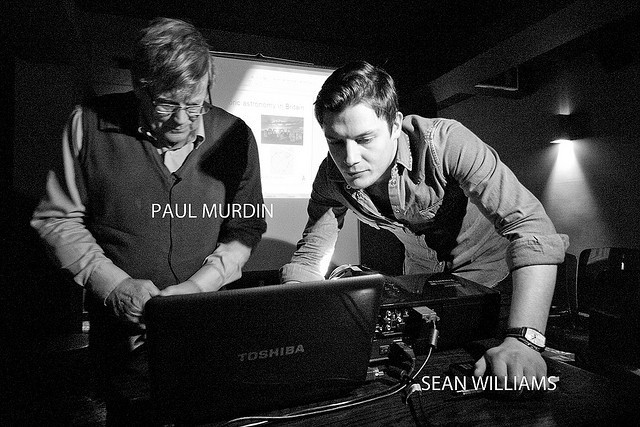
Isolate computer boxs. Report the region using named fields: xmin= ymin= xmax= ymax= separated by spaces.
xmin=368 ymin=272 xmax=500 ymax=362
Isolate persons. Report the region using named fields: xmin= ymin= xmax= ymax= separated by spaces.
xmin=28 ymin=19 xmax=266 ymax=425
xmin=277 ymin=62 xmax=570 ymax=397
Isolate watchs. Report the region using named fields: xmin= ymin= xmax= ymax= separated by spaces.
xmin=505 ymin=326 xmax=546 ymax=353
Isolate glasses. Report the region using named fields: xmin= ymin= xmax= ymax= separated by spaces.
xmin=145 ymin=80 xmax=212 ymax=116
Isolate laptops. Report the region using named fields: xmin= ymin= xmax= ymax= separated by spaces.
xmin=144 ymin=273 xmax=386 ymax=420
